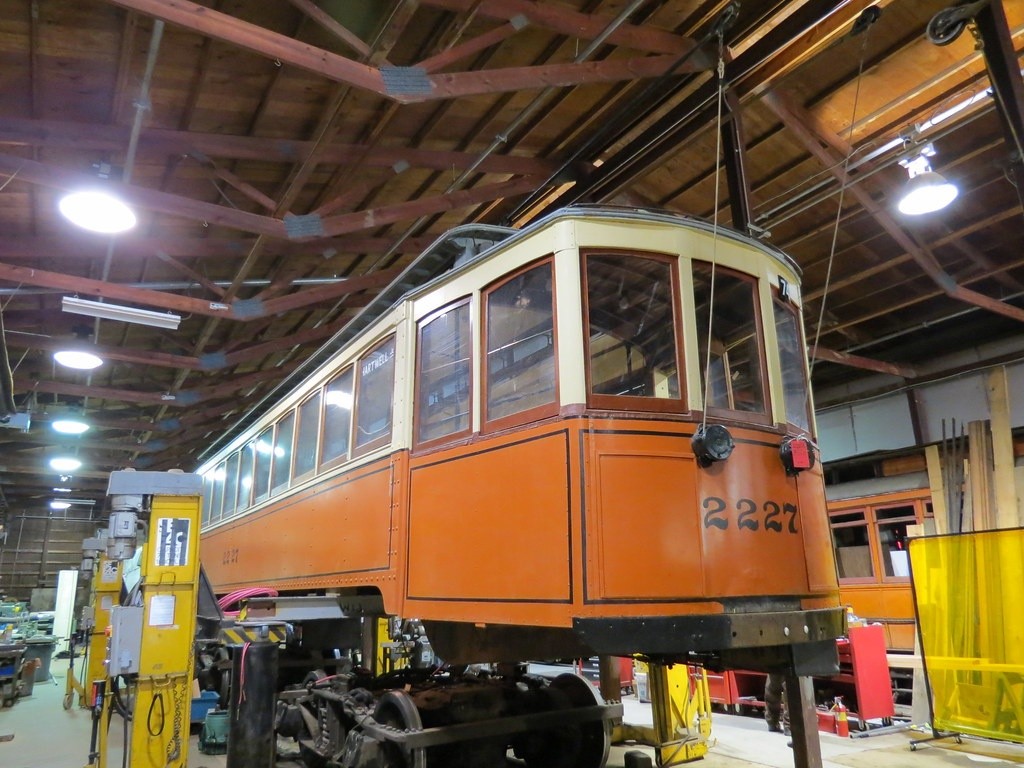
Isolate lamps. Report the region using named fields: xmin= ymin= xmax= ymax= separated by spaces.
xmin=53 ymin=325 xmax=104 ymax=370
xmin=53 ymin=400 xmax=90 ymax=435
xmin=59 ymin=161 xmax=137 ymax=235
xmin=49 ymin=445 xmax=82 ymax=472
xmin=895 ymin=122 xmax=958 ymax=216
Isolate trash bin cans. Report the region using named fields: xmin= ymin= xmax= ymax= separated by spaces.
xmin=23 ymin=634 xmax=57 ymax=683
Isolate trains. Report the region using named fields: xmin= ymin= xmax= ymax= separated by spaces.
xmin=810 ymin=486 xmax=962 ymax=712
xmin=132 ymin=198 xmax=848 ymax=768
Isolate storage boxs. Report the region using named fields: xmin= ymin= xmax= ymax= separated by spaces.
xmin=191 ymin=691 xmax=220 ymax=720
xmin=0 ymin=665 xmax=14 ymax=676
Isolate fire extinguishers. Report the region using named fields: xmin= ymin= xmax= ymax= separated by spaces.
xmin=833 ymin=696 xmax=849 ymax=737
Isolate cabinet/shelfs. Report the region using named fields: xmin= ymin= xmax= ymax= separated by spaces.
xmin=0 ymin=647 xmax=28 ymax=706
xmin=730 ymin=623 xmax=894 ymax=731
